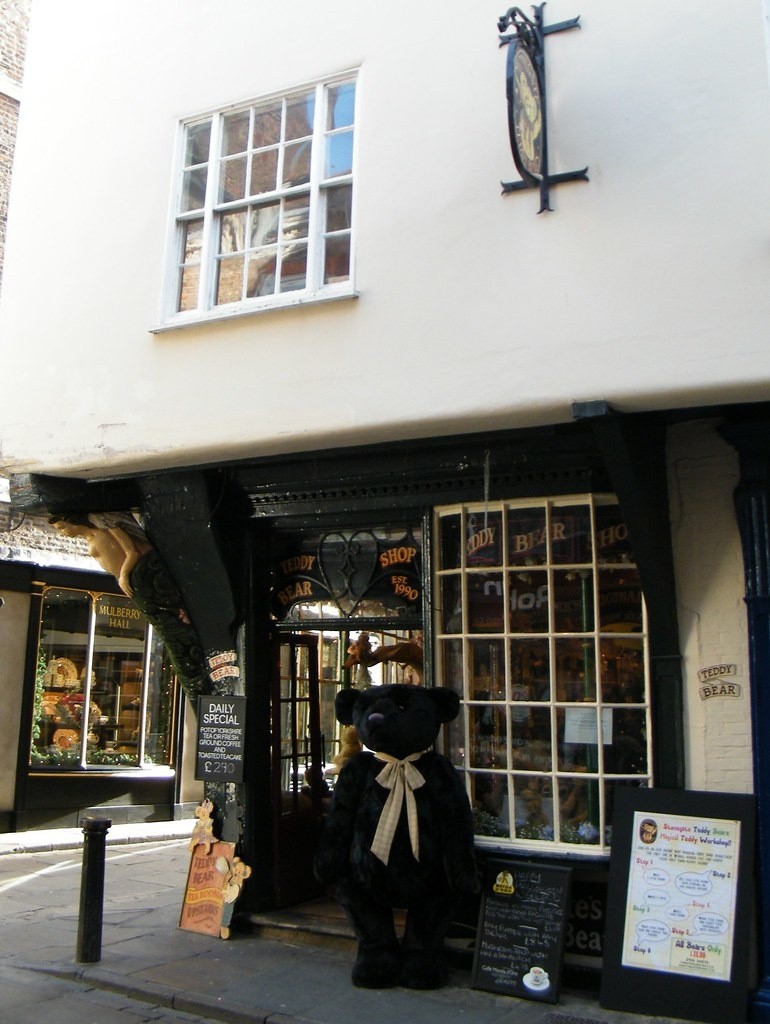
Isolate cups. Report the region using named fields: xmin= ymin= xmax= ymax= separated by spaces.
xmin=98 ymin=716 xmax=108 ymax=726
xmin=53 ymin=674 xmax=64 ymax=688
xmin=105 ymin=741 xmax=117 ymax=751
xmin=42 ymin=674 xmax=51 ymax=687
xmin=87 ymin=733 xmax=99 ymax=744
xmin=65 ymin=679 xmax=74 ymax=687
xmin=530 ymin=967 xmax=548 ymax=985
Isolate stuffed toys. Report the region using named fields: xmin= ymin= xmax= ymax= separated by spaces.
xmin=318 ymin=683 xmax=476 ymax=990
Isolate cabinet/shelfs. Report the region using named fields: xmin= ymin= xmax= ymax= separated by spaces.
xmin=45 ymin=649 xmax=157 ymax=753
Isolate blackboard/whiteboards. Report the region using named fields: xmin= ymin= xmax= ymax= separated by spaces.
xmin=470 ymin=857 xmax=573 ymax=1004
xmin=194 ymin=694 xmax=247 ymax=784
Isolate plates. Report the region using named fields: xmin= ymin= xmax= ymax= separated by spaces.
xmin=524 ymin=973 xmax=550 ymax=990
